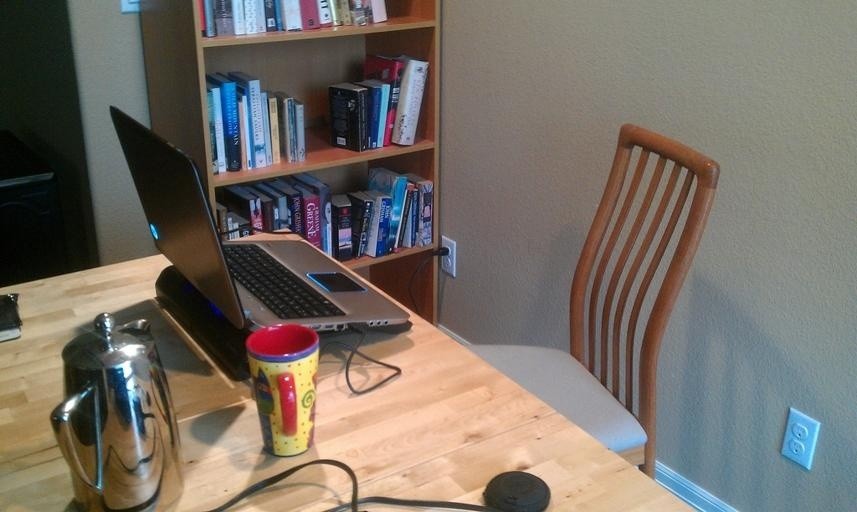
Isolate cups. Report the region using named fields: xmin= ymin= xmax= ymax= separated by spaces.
xmin=244 ymin=323 xmax=321 ymax=458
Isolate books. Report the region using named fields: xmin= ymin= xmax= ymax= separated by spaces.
xmin=328 ymin=53 xmax=430 ymax=152
xmin=199 ymin=1 xmax=388 ymax=38
xmin=215 ymin=167 xmax=433 ymax=262
xmin=205 ymin=71 xmax=306 ymax=176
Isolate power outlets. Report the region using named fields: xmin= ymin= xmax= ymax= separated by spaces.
xmin=440 ymin=236 xmax=457 ymax=278
xmin=780 ymin=406 xmax=822 ymax=471
xmin=463 ymin=123 xmax=720 ymax=481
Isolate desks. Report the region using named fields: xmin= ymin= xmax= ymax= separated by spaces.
xmin=0 ymin=228 xmax=700 ymax=512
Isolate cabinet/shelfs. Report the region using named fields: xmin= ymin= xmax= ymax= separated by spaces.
xmin=137 ymin=0 xmax=440 ymax=329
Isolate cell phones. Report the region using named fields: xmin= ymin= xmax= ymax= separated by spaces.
xmin=0 ymin=295 xmax=21 ymax=330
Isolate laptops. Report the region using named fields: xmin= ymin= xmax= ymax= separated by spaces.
xmin=110 ymin=105 xmax=411 ymax=333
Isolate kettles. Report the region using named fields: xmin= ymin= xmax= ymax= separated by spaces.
xmin=46 ymin=310 xmax=186 ymax=511
